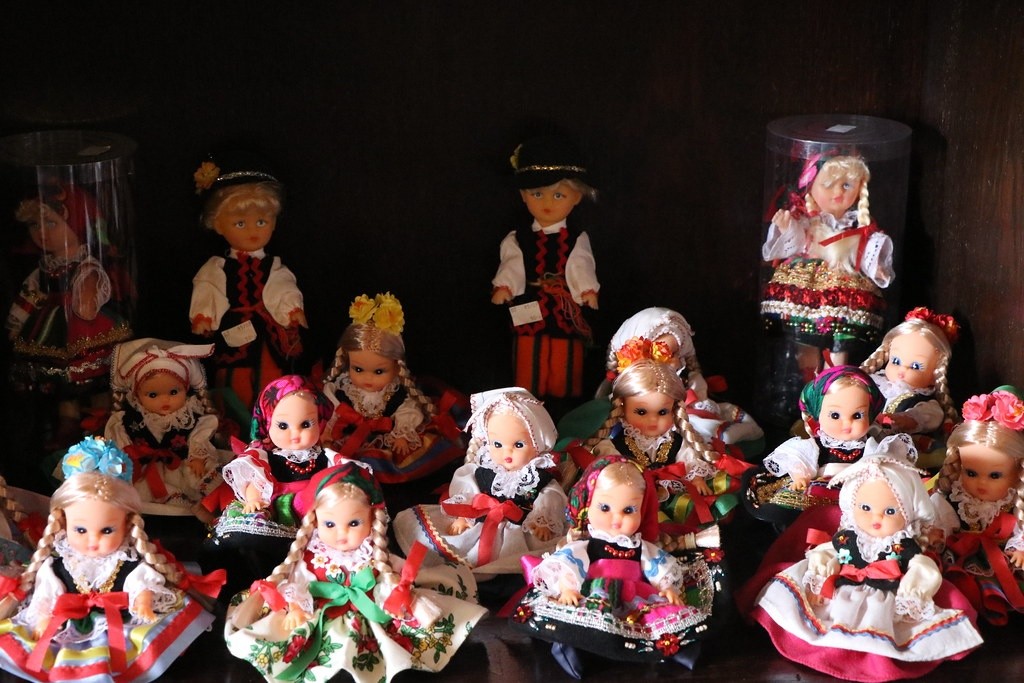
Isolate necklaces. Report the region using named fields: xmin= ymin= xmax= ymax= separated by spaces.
xmin=286 ymin=459 xmax=314 ymax=473
xmin=830 ymin=449 xmax=861 ymax=460
xmin=604 ymin=545 xmax=636 ymax=558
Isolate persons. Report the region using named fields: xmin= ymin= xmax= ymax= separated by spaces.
xmin=761 ymin=149 xmax=894 ymax=385
xmin=492 ymin=136 xmax=601 ymax=423
xmin=0 ymin=437 xmax=227 ymax=683
xmin=0 ymin=154 xmax=464 ymax=567
xmin=393 ymin=386 xmax=567 ymax=583
xmin=859 ymin=308 xmax=957 ymax=469
xmin=751 ymin=433 xmax=985 ymax=683
xmin=556 ymin=307 xmax=764 ymax=537
xmin=226 ymin=462 xmax=490 ymax=683
xmin=922 ymin=386 xmax=1024 ymax=627
xmin=764 ymin=365 xmax=886 ymax=503
xmin=512 ymin=456 xmax=714 ymax=679
